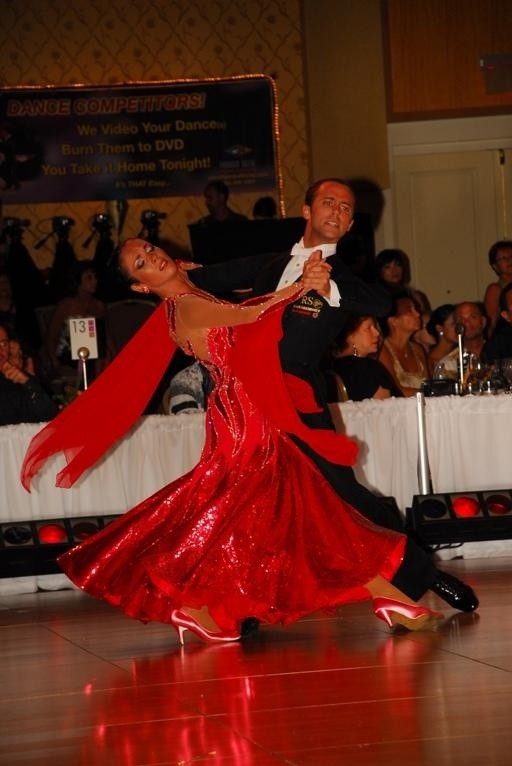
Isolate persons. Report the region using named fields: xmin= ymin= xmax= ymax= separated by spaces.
xmin=22 ymin=235 xmax=448 ymax=647
xmin=1 ymin=180 xmax=511 ymax=420
xmin=221 ymin=177 xmax=478 ymax=637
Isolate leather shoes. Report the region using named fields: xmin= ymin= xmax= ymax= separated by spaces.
xmin=428 ymin=569 xmax=479 ymax=612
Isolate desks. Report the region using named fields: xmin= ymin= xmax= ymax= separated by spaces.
xmin=3 ymin=390 xmax=510 ymax=560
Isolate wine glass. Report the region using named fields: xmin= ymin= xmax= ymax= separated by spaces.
xmin=461 ymin=351 xmax=512 ymax=397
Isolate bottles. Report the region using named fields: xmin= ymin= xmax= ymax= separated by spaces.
xmin=433 ymin=361 xmax=449 ymax=381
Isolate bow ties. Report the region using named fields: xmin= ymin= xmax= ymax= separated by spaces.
xmin=290 ymin=242 xmax=312 ymax=261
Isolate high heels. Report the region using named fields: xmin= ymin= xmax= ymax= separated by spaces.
xmin=371 ymin=596 xmax=444 ymax=632
xmin=170 ymin=608 xmax=242 ymax=645
